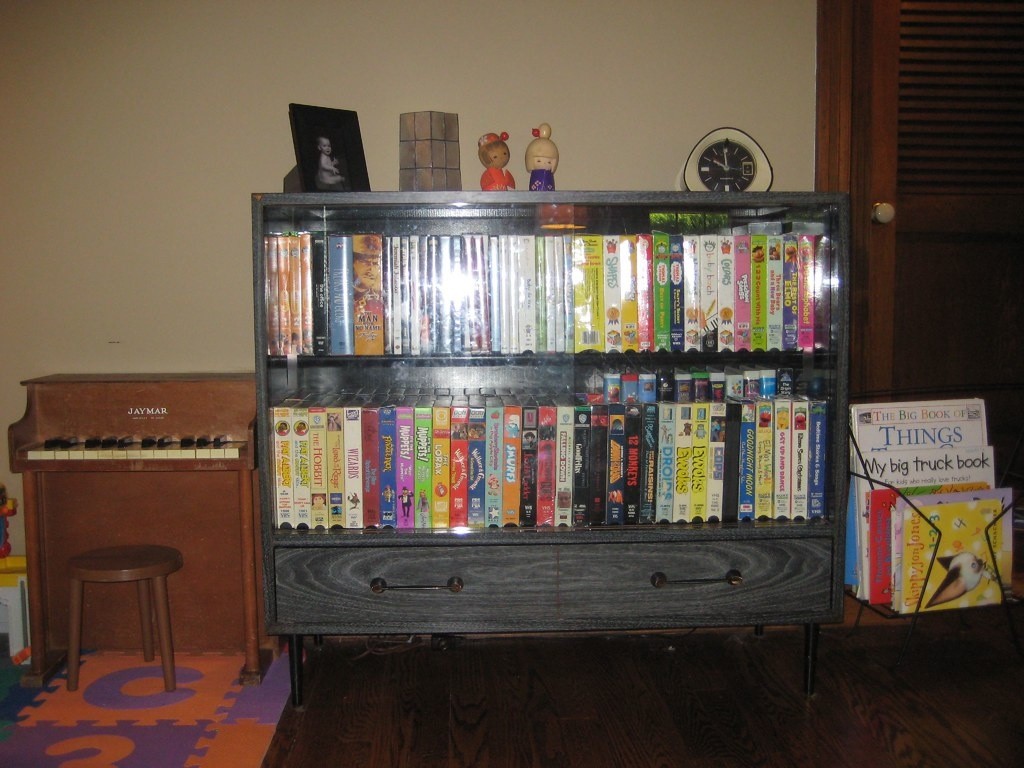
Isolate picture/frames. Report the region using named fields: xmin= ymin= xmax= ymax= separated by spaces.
xmin=288 ymin=103 xmax=370 ymax=192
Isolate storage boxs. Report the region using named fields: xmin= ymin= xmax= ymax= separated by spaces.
xmin=0 ymin=556 xmax=31 ymax=665
xmin=271 ymin=364 xmax=826 ymax=531
xmin=264 ymin=230 xmax=834 ymax=357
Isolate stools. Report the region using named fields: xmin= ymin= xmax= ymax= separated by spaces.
xmin=67 ymin=543 xmax=184 ymax=692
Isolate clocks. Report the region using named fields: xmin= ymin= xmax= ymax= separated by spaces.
xmin=683 ymin=128 xmax=774 ymax=193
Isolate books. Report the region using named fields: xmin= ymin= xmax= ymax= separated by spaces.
xmin=263 ymin=225 xmax=1016 ymax=613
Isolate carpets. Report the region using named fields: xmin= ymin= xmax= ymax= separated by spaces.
xmin=0 ymin=649 xmax=307 ymax=768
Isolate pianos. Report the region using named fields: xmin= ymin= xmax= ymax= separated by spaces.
xmin=9 ymin=371 xmax=271 ymax=687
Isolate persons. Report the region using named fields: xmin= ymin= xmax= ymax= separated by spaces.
xmin=318 ymin=137 xmax=347 ymax=185
xmin=480 ymin=125 xmax=558 ymax=191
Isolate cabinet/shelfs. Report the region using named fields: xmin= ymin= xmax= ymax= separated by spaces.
xmin=250 ymin=191 xmax=852 ymax=711
